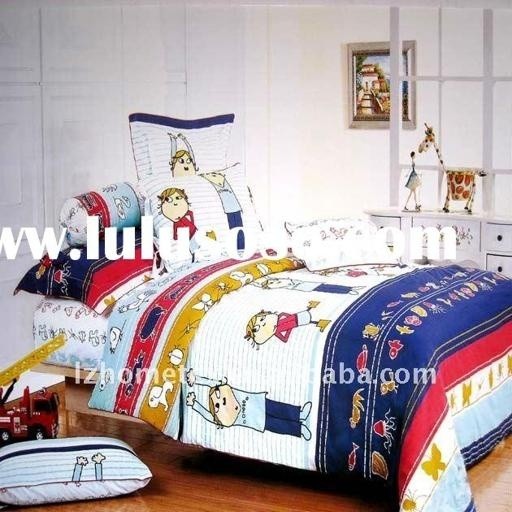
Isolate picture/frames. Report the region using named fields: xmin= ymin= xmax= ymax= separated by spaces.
xmin=347 ymin=40 xmax=417 ymax=130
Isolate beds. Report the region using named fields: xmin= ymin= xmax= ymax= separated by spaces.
xmin=31 ymin=250 xmax=512 ymax=512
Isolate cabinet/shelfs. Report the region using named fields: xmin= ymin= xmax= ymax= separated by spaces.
xmin=363 ymin=208 xmax=512 ymax=278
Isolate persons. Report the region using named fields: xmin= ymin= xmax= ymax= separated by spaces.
xmin=0 ymin=332 xmax=68 ymax=444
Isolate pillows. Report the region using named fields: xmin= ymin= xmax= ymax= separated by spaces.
xmin=13 ymin=113 xmax=399 ymax=314
xmin=0 ymin=437 xmax=153 ymax=507
